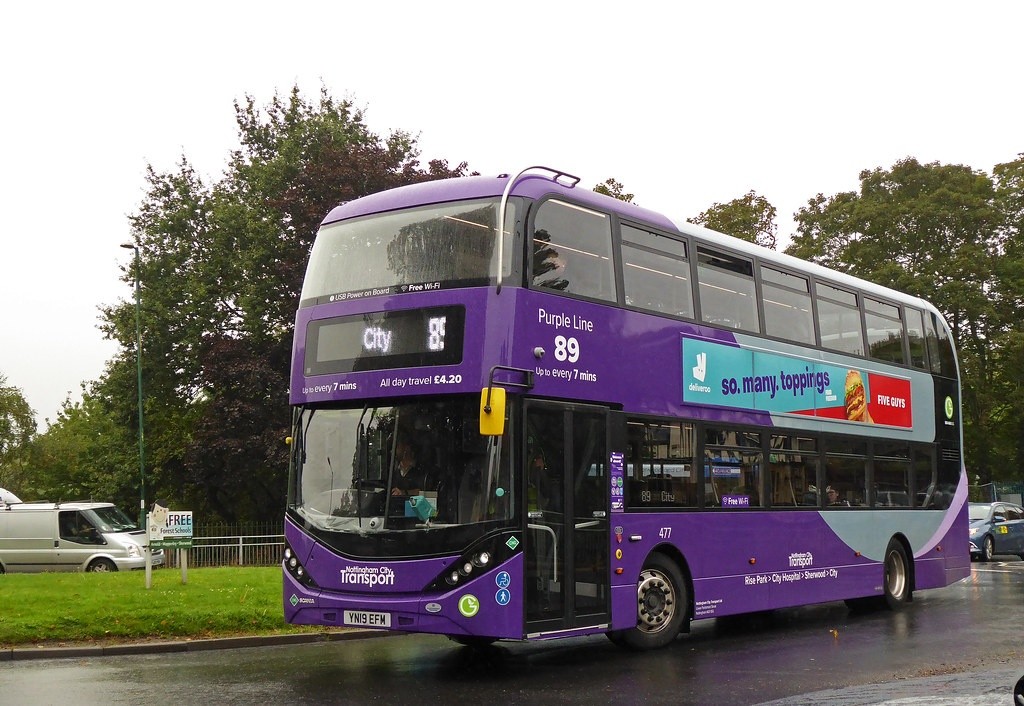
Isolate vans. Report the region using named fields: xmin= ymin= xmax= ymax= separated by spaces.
xmin=0 ymin=487 xmax=166 ymax=572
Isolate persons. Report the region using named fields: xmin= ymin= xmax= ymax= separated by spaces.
xmin=823 ymin=484 xmax=843 ymax=505
xmin=362 ymin=436 xmax=434 ymax=519
xmin=537 ymin=246 xmax=574 ymax=290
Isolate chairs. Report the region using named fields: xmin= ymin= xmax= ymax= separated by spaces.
xmin=632 ymin=472 xmax=927 ymax=509
xmin=835 ymin=337 xmax=931 ymax=370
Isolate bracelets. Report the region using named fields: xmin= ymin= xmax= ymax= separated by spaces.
xmin=404 ymin=490 xmax=409 ymax=495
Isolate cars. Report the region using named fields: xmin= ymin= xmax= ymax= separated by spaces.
xmin=967 ymin=501 xmax=1024 ymax=561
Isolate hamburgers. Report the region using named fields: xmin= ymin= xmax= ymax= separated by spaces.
xmin=845 ymin=370 xmax=866 ymax=422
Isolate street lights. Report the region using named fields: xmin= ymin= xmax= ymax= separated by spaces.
xmin=119 ymin=241 xmax=153 ymax=588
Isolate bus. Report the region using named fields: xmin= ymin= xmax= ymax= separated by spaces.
xmin=281 ymin=165 xmax=971 ymax=651
